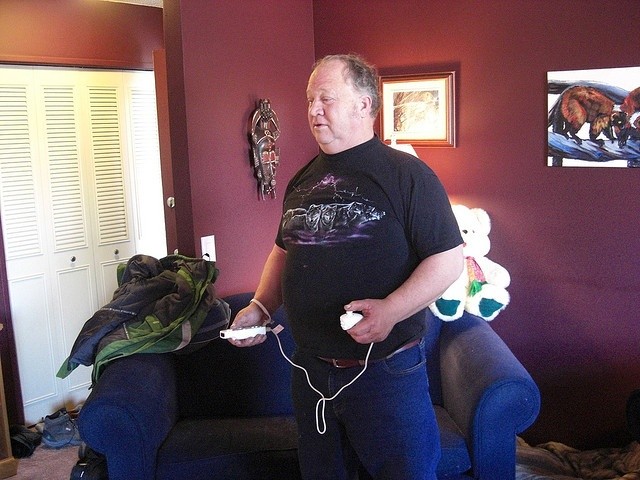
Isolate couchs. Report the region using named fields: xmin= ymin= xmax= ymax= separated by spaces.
xmin=79 ymin=293 xmax=540 ymax=480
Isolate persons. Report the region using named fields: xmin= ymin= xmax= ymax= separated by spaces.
xmin=225 ymin=53 xmax=466 ymax=479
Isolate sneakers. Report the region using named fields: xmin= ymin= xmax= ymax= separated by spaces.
xmin=45 ymin=404 xmax=83 ymax=430
xmin=42 ymin=419 xmax=83 ymax=448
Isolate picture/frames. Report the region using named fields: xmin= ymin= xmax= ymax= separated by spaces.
xmin=378 ymin=71 xmax=458 ymax=149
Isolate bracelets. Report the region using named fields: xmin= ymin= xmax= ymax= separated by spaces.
xmin=247 ymin=295 xmax=276 ymax=324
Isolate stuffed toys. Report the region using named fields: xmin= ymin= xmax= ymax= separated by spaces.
xmin=426 ymin=204 xmax=511 ymax=323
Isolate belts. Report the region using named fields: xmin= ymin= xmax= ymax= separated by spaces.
xmin=316 ymin=340 xmax=420 ymax=370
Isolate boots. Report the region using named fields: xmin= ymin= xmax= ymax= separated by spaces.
xmin=9 ymin=424 xmax=41 ymax=459
